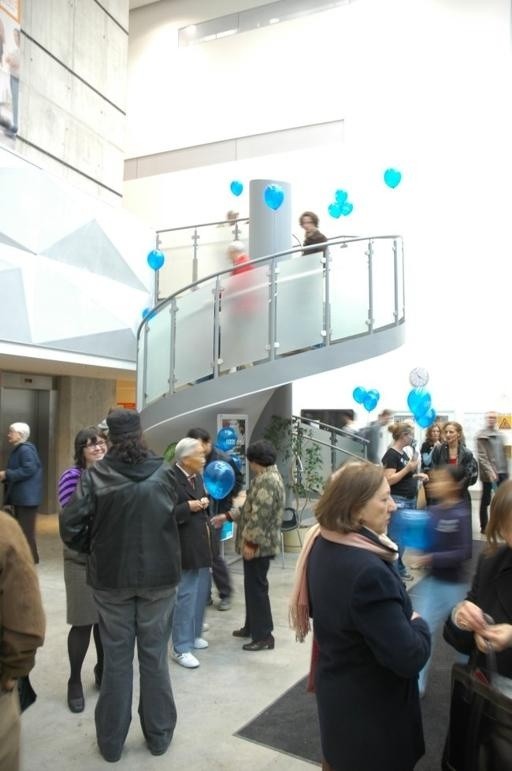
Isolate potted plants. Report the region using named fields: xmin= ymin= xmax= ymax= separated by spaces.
xmin=261 ymin=415 xmax=326 ymax=553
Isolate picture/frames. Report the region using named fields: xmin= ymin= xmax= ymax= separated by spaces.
xmin=216 ymin=413 xmax=250 ymax=491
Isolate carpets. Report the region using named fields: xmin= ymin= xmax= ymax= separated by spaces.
xmin=232 ymin=540 xmax=501 ymax=771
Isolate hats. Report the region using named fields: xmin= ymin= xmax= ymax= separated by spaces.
xmin=107 ymin=408 xmax=141 ymax=433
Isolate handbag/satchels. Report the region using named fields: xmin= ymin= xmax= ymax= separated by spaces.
xmin=441 ymin=613 xmax=512 ymax=771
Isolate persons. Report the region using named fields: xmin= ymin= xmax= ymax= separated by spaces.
xmin=288 ymin=459 xmax=432 ymax=771
xmin=167 ymin=428 xmax=286 ymax=670
xmin=0 ymin=509 xmax=45 ymax=771
xmin=58 ymin=405 xmax=181 ymax=763
xmin=295 ymin=208 xmax=331 ymax=353
xmin=220 ymin=238 xmax=265 ymax=375
xmin=440 ymin=479 xmax=512 ymax=771
xmin=0 ymin=422 xmax=39 ymax=564
xmin=55 ymin=425 xmax=106 ymax=716
xmin=343 ymin=410 xmax=512 ymax=699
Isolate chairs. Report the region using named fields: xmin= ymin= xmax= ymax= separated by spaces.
xmin=279 ymin=507 xmax=303 ymax=569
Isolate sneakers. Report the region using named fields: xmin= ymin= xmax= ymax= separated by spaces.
xmin=219 ymin=598 xmax=231 ymax=610
xmin=173 ymin=623 xmax=209 ymax=668
xmin=400 ymin=571 xmax=413 ymax=581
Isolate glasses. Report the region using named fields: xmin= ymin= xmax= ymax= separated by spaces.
xmin=85 ymin=441 xmax=105 ymax=449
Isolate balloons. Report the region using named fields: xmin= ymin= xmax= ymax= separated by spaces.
xmin=142 ymin=307 xmax=159 ymax=322
xmin=327 ymin=202 xmax=341 ymax=219
xmin=231 ymin=179 xmax=244 ymax=197
xmin=383 ymin=168 xmax=402 ymax=189
xmin=408 ymin=388 xmax=433 ymax=418
xmin=262 ymin=183 xmax=285 ymax=212
xmin=335 ymin=189 xmax=348 ymax=204
xmin=363 ymin=395 xmax=378 ymax=412
xmin=340 ymin=202 xmax=353 ymax=216
xmin=353 ymin=386 xmax=365 ymax=403
xmin=147 ymin=249 xmax=165 ymax=271
xmin=367 ymin=388 xmax=381 ymax=402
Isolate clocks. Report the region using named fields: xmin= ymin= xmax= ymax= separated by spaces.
xmin=409 ymin=368 xmax=429 ymax=387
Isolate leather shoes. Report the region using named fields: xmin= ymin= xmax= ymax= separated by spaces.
xmin=233 ymin=628 xmax=248 ymax=637
xmin=243 ymin=635 xmax=274 ymax=650
xmin=68 ymin=680 xmax=84 ymax=712
